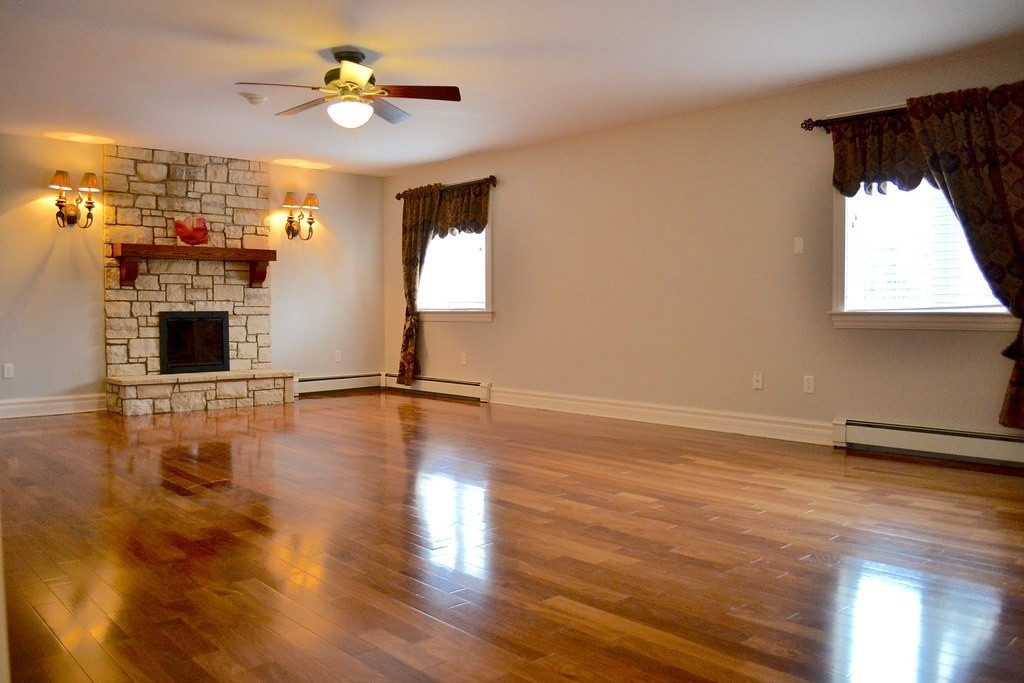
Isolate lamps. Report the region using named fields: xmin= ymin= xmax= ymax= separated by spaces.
xmin=282 ymin=190 xmax=320 ymax=240
xmin=326 ymin=100 xmax=374 ymax=130
xmin=48 ymin=169 xmax=101 ymax=231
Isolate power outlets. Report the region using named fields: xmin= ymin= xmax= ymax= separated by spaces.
xmin=752 ymin=371 xmax=763 ymax=390
xmin=335 ymin=351 xmax=342 ymax=362
xmin=803 ymin=374 xmax=814 ymax=395
xmin=3 ymin=363 xmax=14 ymax=378
xmin=460 ymin=352 xmax=467 ymax=366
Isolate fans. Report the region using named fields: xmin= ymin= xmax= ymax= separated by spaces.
xmin=234 ymin=49 xmax=463 ymax=125
